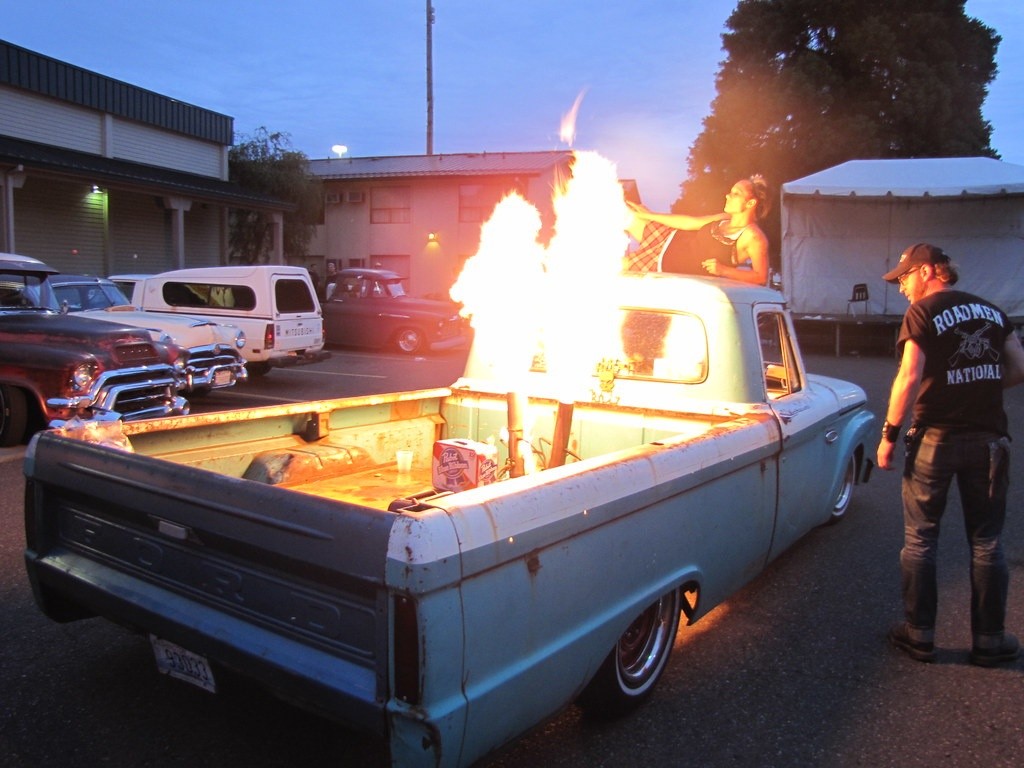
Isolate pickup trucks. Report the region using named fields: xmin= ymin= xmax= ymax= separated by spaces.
xmin=319 ymin=268 xmax=467 ymax=354
xmin=19 ymin=272 xmax=874 ymax=768
xmin=107 ymin=266 xmax=331 ymax=378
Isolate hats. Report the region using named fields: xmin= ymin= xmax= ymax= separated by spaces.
xmin=881 ymin=243 xmax=947 ymax=284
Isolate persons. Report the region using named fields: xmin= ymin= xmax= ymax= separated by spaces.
xmin=326 ymin=262 xmax=342 ymax=284
xmin=623 ymin=173 xmax=771 ymax=287
xmin=875 ymin=244 xmax=1024 ymax=668
xmin=308 ymin=263 xmax=321 ymax=293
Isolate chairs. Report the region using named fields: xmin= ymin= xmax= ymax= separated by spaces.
xmin=846 ymin=283 xmax=867 ymax=317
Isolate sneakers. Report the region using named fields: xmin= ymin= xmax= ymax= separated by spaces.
xmin=971 ymin=631 xmax=1020 ymax=665
xmin=889 ymin=622 xmax=936 ymax=662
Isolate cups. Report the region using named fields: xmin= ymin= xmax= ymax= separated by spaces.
xmin=397 ymin=451 xmax=413 ymax=473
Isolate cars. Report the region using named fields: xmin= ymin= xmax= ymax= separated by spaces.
xmin=34 ymin=274 xmax=249 ymax=395
xmin=0 ymin=304 xmax=190 ymax=448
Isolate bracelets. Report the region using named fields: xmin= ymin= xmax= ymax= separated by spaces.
xmin=882 ymin=419 xmax=903 ymax=444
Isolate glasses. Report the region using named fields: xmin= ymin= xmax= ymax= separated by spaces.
xmin=894 ymin=267 xmax=924 ymax=286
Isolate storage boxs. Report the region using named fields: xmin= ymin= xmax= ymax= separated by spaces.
xmin=430 ymin=437 xmax=497 ymax=493
